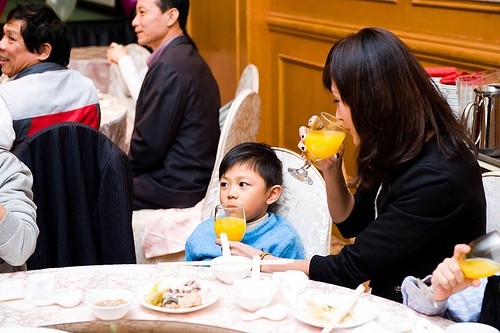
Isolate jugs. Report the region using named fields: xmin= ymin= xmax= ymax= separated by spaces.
xmin=460 ymin=83 xmax=500 ymax=156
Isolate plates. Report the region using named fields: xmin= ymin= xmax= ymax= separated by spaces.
xmin=292 ymin=292 xmax=378 ymax=328
xmin=140 ymin=279 xmax=219 ymax=313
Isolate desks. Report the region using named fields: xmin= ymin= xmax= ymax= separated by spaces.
xmin=48 ymin=7 xmax=122 ymax=48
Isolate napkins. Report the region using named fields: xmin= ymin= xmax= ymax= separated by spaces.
xmin=236 ymin=270 xmax=308 ymax=311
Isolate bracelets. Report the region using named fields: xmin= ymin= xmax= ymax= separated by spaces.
xmin=259 ymin=252 xmax=270 ymax=260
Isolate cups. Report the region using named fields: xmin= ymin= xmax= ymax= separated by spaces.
xmin=455 ymin=74 xmax=477 ymax=134
xmin=214 ymin=204 xmax=246 ymax=242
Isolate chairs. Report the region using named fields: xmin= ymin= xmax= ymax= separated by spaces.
xmin=10 ymin=44 xmax=332 ymax=272
xmin=480 ymin=169 xmax=500 ymax=235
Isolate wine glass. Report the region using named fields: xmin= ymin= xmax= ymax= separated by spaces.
xmin=288 ymin=112 xmax=349 ymax=185
xmin=417 ymin=230 xmax=500 ymax=308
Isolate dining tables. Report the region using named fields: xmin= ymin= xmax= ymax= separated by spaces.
xmin=66 ymin=43 xmax=110 ymax=94
xmin=0 ymin=264 xmax=500 ymax=333
xmin=85 ymin=89 xmax=129 ymax=156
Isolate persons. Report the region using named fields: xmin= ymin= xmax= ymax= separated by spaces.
xmin=128 ymin=0 xmax=221 ymax=211
xmin=215 ymin=27 xmax=487 ymax=304
xmin=106 ymin=42 xmax=142 ymax=102
xmin=401 ymin=244 xmax=500 ymax=330
xmin=0 ymin=0 xmax=101 ymax=153
xmin=0 ymin=148 xmax=40 ymax=266
xmin=184 ymin=142 xmax=305 ymax=262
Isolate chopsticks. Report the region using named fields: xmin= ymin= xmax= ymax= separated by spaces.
xmin=321 ymin=284 xmax=365 ymax=333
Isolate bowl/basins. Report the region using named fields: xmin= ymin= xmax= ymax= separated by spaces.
xmin=87 ymin=289 xmax=134 ymax=320
xmin=211 ymin=256 xmax=252 ymax=284
xmin=233 ymin=278 xmax=274 ymax=310
xmin=445 ymin=322 xmax=500 ymax=333
